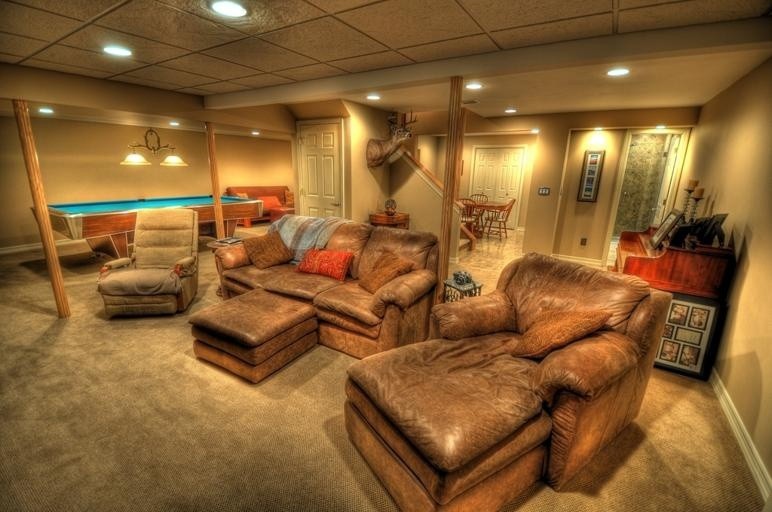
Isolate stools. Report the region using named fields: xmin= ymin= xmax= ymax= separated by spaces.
xmin=187 ymin=289 xmax=319 ymax=383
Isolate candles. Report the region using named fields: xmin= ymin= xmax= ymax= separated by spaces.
xmin=686 ymin=177 xmax=698 ymax=190
xmin=692 ymin=186 xmax=705 ymax=200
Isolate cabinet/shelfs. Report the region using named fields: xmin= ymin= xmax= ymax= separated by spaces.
xmin=370 ymin=211 xmax=410 ymax=229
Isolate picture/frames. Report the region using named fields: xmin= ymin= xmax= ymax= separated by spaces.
xmin=650 ymin=207 xmax=684 ymax=249
xmin=576 ymin=148 xmax=606 ymax=204
xmin=652 ymin=289 xmax=726 ymax=383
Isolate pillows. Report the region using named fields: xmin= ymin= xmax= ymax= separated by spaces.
xmin=236 ymin=192 xmax=249 ymax=198
xmin=241 ymin=230 xmax=295 ymax=270
xmin=295 ymin=249 xmax=355 ymax=282
xmin=358 ymin=245 xmax=416 ymax=294
xmin=511 ymin=309 xmax=614 ymax=360
xmin=257 ymin=195 xmax=283 ymax=209
xmin=284 ymin=190 xmax=294 ymax=205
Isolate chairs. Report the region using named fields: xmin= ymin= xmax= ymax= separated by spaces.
xmin=339 ymin=251 xmax=676 ymax=512
xmin=457 ymin=194 xmax=517 ymax=242
xmin=95 ymin=205 xmax=199 ymax=317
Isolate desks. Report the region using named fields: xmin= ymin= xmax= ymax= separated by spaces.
xmin=270 ymin=205 xmax=295 ymax=224
xmin=441 ymin=278 xmax=483 ymax=303
xmin=29 ymin=195 xmax=269 ymax=263
xmin=205 ymin=237 xmax=247 ymax=297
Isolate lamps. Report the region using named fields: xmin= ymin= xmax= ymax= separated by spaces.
xmin=118 ymin=125 xmax=188 ymax=169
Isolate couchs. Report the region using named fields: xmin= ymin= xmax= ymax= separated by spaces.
xmin=223 ymin=185 xmax=294 ymax=227
xmin=212 ymin=214 xmax=440 ymax=361
